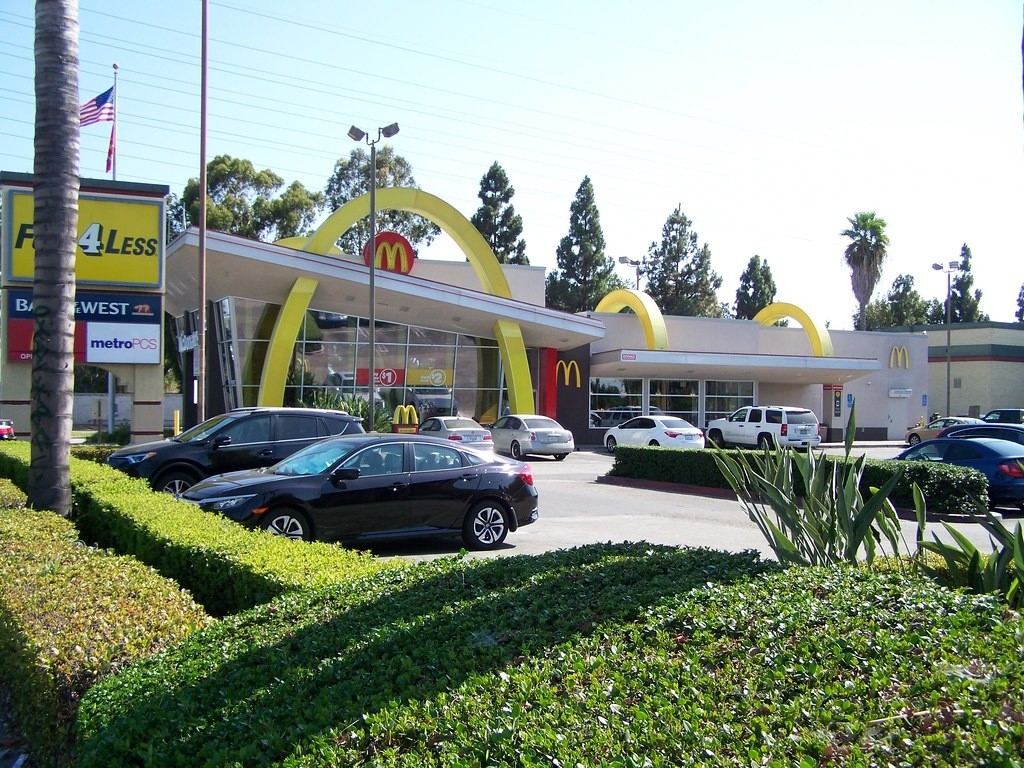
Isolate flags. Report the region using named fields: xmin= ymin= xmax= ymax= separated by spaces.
xmin=106 ymin=125 xmax=116 ymax=172
xmin=80 ymin=84 xmax=115 ymax=126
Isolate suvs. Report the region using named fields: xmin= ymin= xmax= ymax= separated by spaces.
xmin=107 ymin=407 xmax=367 ymax=503
xmin=705 ymin=404 xmax=821 ymax=453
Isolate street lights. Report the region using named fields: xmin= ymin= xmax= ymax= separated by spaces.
xmin=347 ymin=123 xmax=400 ymax=435
xmin=619 ymin=257 xmax=658 ymax=291
xmin=932 ymin=261 xmax=958 ymax=417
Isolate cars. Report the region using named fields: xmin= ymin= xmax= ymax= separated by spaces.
xmin=484 ymin=414 xmax=574 ymax=461
xmin=317 ymin=311 xmax=347 ymax=328
xmin=885 ymin=409 xmax=1024 ymax=512
xmin=419 ymin=416 xmax=494 ymax=450
xmin=389 ymin=384 xmax=459 ymax=418
xmin=590 ymin=406 xmax=664 ymax=428
xmin=182 ymin=433 xmax=539 ymax=551
xmin=323 ymin=371 xmax=385 ymax=409
xmin=604 ymin=416 xmax=705 ymax=453
